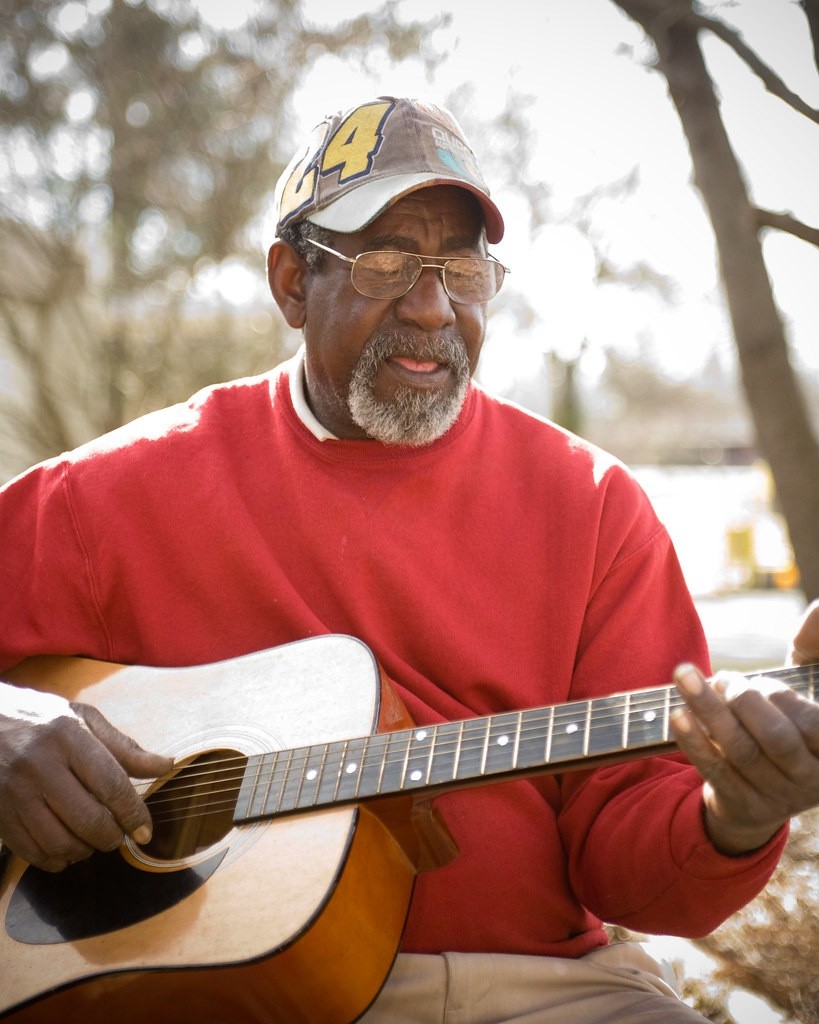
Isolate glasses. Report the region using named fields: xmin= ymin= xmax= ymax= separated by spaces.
xmin=295 ymin=231 xmax=513 ymax=306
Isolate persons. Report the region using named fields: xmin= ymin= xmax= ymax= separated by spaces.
xmin=2 ymin=96 xmax=817 ymax=1021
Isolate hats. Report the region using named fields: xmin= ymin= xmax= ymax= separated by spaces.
xmin=272 ymin=94 xmax=505 ymax=245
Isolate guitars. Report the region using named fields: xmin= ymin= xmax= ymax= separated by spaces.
xmin=0 ymin=633 xmax=819 ymax=1024
